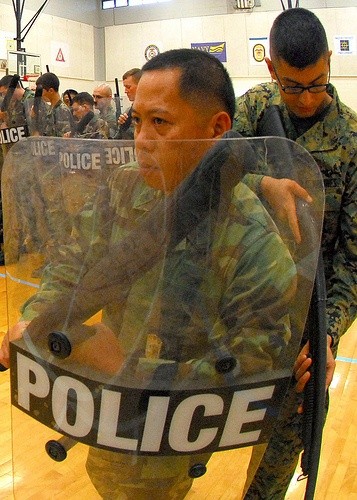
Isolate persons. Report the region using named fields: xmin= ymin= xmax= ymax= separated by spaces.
xmin=0 ymin=47 xmax=301 ymax=500
xmin=0 ymin=66 xmax=142 ymax=279
xmin=216 ymin=6 xmax=357 ymax=500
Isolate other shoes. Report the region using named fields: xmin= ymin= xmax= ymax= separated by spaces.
xmin=31 ymin=266 xmax=44 ymax=278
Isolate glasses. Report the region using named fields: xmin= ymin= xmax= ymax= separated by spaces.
xmin=274 ymin=59 xmax=330 ymax=94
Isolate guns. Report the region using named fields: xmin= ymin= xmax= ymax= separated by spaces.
xmin=256 ymin=103 xmax=326 ymax=498
xmin=0 ymin=131 xmax=257 ymax=373
xmin=0 ymin=62 xmax=135 ymax=134
